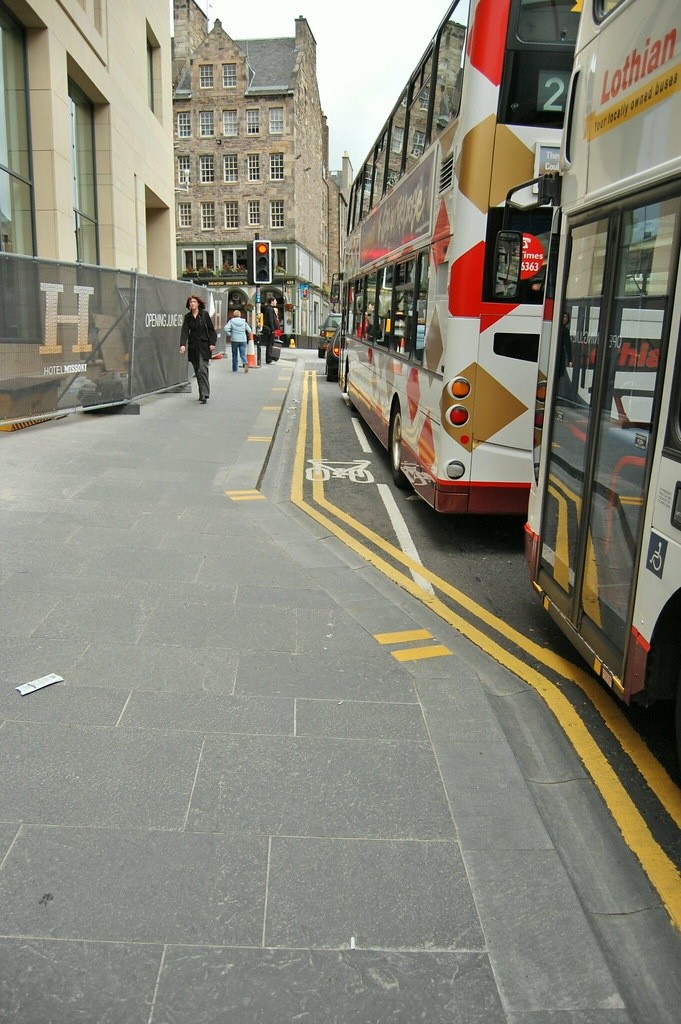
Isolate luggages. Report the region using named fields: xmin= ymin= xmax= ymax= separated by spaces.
xmin=271 ymin=334 xmax=282 ymax=361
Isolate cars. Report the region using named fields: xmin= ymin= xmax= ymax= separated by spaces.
xmin=322 ymin=326 xmax=343 ymax=381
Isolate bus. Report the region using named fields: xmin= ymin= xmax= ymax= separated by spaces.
xmin=329 ymin=0 xmax=681 ymax=510
xmin=503 ymin=0 xmax=681 ymax=751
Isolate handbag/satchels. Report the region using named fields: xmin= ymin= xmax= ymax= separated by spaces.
xmin=262 ymin=325 xmax=271 ymax=335
xmin=376 ymin=328 xmax=383 ymax=339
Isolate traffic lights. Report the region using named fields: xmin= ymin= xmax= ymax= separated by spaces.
xmin=252 ymin=240 xmax=273 ymax=285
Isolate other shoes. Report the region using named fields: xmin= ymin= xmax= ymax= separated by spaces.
xmin=201 ymin=396 xmax=207 ymax=403
xmin=245 ymin=364 xmax=249 ymax=373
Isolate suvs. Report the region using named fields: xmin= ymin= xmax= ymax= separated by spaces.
xmin=318 ymin=313 xmax=352 ymax=359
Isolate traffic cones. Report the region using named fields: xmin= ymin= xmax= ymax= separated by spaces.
xmin=244 ymin=331 xmax=261 ymax=369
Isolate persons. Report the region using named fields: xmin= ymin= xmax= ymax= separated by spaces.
xmin=263 ymin=296 xmax=280 ymax=365
xmin=223 ymin=310 xmax=251 ymax=373
xmin=552 ymin=311 xmax=573 ymax=396
xmin=179 ymin=295 xmax=217 ymax=404
xmin=367 ymin=300 xmax=383 ymax=341
